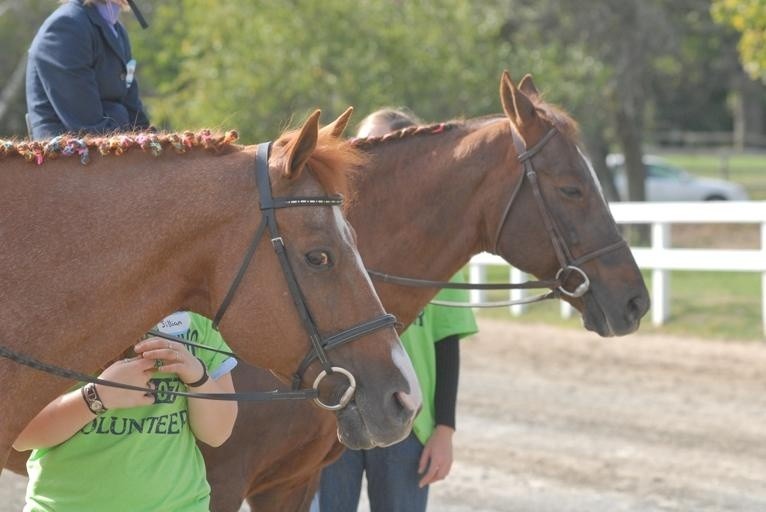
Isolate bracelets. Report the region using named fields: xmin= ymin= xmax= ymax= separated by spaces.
xmin=79 ymin=382 xmax=108 ymax=418
xmin=180 ymin=358 xmax=211 ymax=388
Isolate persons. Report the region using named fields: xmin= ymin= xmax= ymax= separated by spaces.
xmin=26 ymin=1 xmax=153 ymax=138
xmin=309 ymin=106 xmax=478 ymax=512
xmin=13 ymin=308 xmax=238 ymax=512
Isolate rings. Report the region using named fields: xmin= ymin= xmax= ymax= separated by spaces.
xmin=166 ymin=338 xmax=174 ymax=349
xmin=124 ymin=358 xmax=129 ymax=363
xmin=175 ymin=349 xmax=179 ymax=361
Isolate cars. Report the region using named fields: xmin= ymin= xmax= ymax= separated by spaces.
xmin=605 ymin=153 xmax=749 ymax=205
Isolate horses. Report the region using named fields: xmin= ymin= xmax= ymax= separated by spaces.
xmin=4 ymin=65 xmax=655 ymax=512
xmin=0 ymin=104 xmax=425 ymax=469
